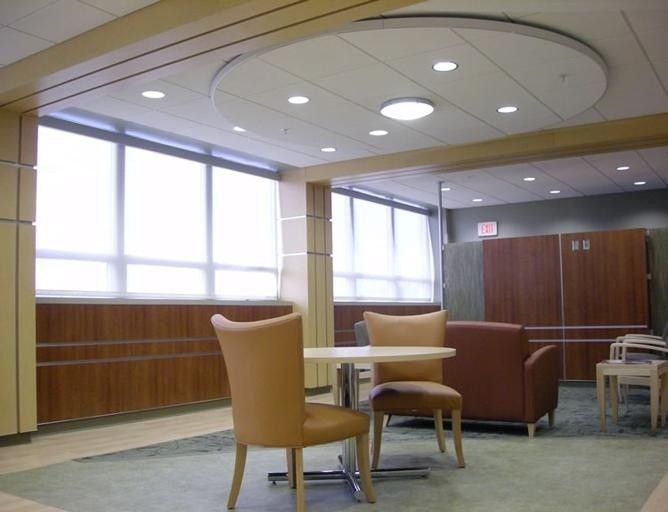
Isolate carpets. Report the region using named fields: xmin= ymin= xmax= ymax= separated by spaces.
xmin=0 ymin=381 xmax=668 ymax=512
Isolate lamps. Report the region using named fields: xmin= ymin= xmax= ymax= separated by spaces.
xmin=379 ymin=97 xmax=434 ymax=122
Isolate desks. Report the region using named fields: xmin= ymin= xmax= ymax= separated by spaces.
xmin=267 ymin=345 xmax=456 ymax=502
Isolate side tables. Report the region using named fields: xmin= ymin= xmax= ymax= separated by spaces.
xmin=595 ymin=360 xmax=668 ymax=432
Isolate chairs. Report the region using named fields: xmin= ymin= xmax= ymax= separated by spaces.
xmin=363 ymin=310 xmax=467 ymax=468
xmin=610 ymin=322 xmax=668 ymax=410
xmin=210 ymin=312 xmax=377 ymax=512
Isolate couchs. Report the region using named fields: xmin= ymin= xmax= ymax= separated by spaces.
xmin=383 ymin=320 xmax=560 ymax=439
